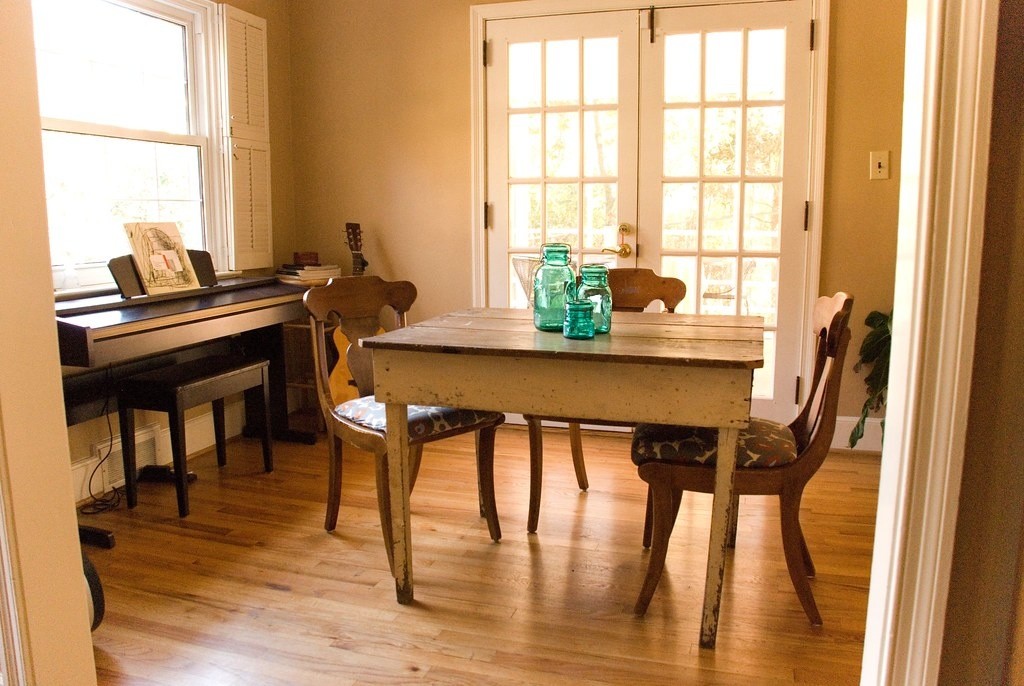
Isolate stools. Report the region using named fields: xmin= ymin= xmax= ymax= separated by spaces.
xmin=113 ymin=356 xmax=274 ymax=516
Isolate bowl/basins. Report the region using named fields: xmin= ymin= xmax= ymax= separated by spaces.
xmin=293 ymin=250 xmax=319 ymax=265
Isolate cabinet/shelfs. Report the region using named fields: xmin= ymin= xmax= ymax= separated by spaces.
xmin=283 ymin=318 xmax=339 ymax=433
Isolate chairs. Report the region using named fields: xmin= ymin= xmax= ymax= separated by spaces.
xmin=303 ymin=275 xmax=506 ymax=543
xmin=524 ymin=268 xmax=687 ymax=532
xmin=632 ymin=291 xmax=855 ymax=625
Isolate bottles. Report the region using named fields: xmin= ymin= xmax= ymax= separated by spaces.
xmin=562 ymin=300 xmax=594 ymax=339
xmin=579 ymin=264 xmax=613 ymax=333
xmin=535 ymin=244 xmax=576 ymax=332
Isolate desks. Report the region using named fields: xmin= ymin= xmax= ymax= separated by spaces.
xmin=358 ymin=304 xmax=763 ymax=649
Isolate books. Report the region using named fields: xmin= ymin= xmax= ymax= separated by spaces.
xmin=123 ymin=221 xmax=201 ymax=295
xmin=275 ymin=263 xmax=341 ymax=288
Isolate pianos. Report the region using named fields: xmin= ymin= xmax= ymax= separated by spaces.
xmin=56 ymin=276 xmax=317 ymax=549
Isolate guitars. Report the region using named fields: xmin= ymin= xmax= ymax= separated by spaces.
xmin=327 ymin=222 xmax=385 ymax=406
